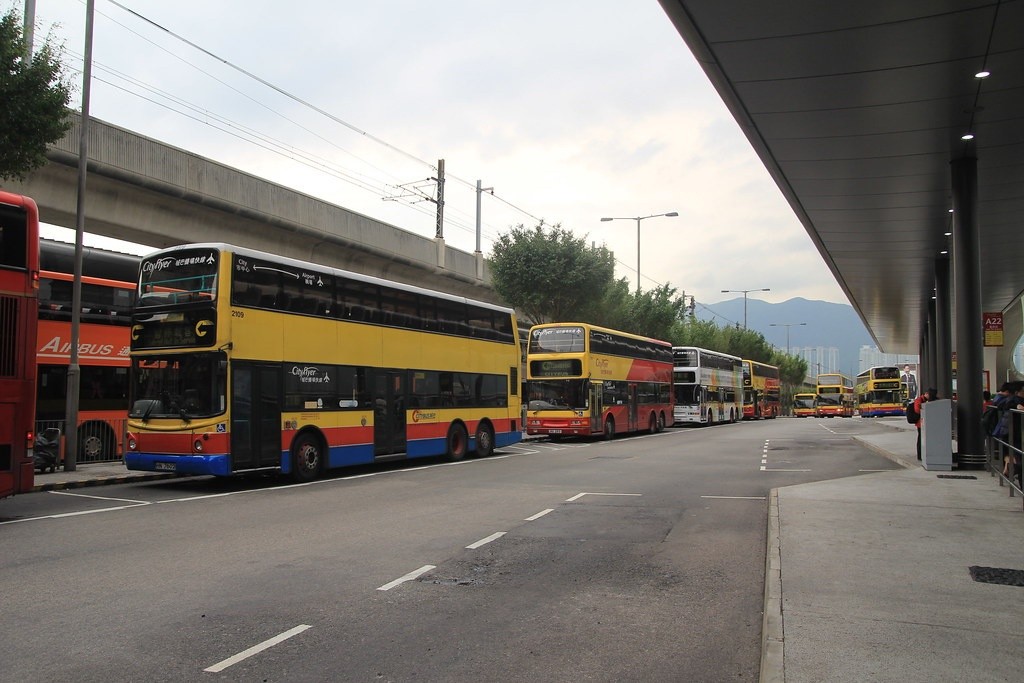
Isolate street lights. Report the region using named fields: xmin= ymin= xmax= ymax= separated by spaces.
xmin=721 ymin=288 xmax=773 ymax=334
xmin=770 ymin=323 xmax=807 ymax=355
xmin=600 ymin=211 xmax=678 ymax=297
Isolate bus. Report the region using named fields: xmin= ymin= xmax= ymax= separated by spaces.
xmin=527 ymin=323 xmax=675 ymax=440
xmin=0 ymin=191 xmax=36 ymax=497
xmin=856 ymin=366 xmax=904 ymax=418
xmin=741 ymin=360 xmax=782 ymax=418
xmin=793 ymin=394 xmax=817 ymax=417
xmin=134 ymin=245 xmax=528 ymax=476
xmin=816 ymin=374 xmax=854 ymax=418
xmin=668 ymin=346 xmax=744 ymax=424
xmin=37 ymin=238 xmax=216 ymax=464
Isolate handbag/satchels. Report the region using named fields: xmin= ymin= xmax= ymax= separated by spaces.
xmin=907 ymin=402 xmax=921 ymax=424
xmin=983 ymin=396 xmax=1006 ymax=435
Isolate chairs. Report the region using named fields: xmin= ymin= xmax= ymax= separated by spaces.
xmin=231 ymin=285 xmax=515 ymax=343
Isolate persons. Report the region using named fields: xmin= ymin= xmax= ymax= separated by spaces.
xmin=901 ymin=364 xmax=915 ymax=382
xmin=914 ymin=387 xmax=930 ymax=461
xmin=983 ymin=380 xmax=1024 ymax=488
